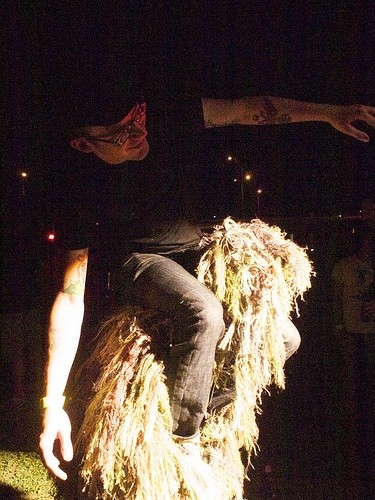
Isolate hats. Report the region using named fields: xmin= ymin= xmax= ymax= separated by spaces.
xmin=55 ymin=80 xmax=144 ymax=130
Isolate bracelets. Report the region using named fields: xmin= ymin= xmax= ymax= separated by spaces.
xmin=40 ymin=396 xmax=67 ymax=409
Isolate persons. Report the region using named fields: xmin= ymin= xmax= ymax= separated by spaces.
xmin=39 ymin=59 xmax=375 ymax=480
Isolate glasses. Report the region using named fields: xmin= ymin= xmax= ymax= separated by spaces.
xmin=83 ymin=96 xmax=147 ymax=146
